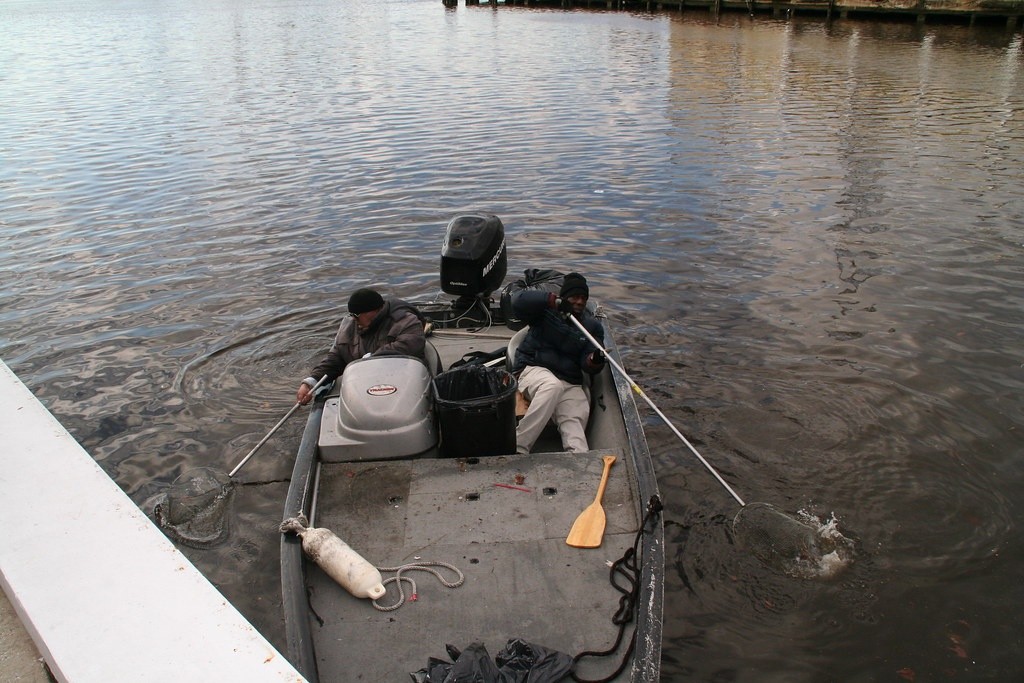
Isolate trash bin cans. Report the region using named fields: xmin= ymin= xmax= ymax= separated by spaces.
xmin=430 ymin=363 xmax=519 ymax=459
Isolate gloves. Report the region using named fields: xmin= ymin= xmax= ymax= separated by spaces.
xmin=586 ymin=346 xmax=613 ymax=369
xmin=554 ymin=296 xmax=574 ymax=319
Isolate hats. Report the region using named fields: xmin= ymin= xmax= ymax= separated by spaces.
xmin=347 ymin=288 xmax=384 ymax=313
xmin=559 ymin=272 xmax=589 ymax=300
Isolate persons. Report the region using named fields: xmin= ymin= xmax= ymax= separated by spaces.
xmin=511 ymin=272 xmax=613 ymax=454
xmin=297 ymin=288 xmax=432 ymax=405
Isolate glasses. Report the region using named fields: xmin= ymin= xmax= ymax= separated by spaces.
xmin=348 ymin=313 xmax=362 ymax=319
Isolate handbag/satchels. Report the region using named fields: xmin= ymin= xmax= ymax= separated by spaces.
xmin=447 ymin=347 xmax=507 ymax=370
